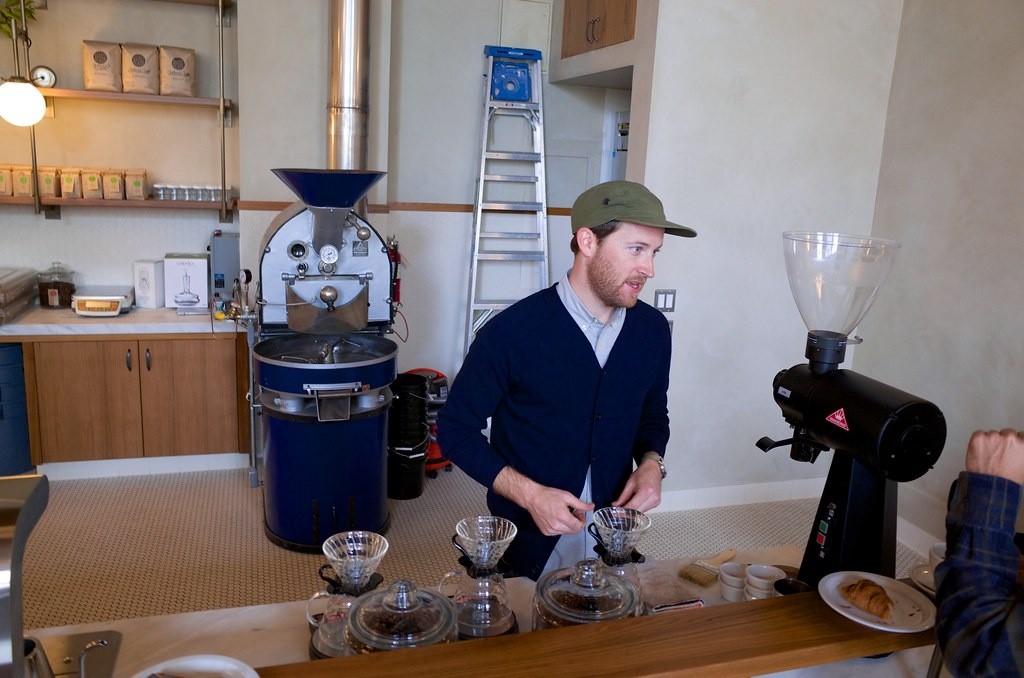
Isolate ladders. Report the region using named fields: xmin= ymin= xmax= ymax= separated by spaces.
xmin=464 ymin=44 xmax=549 ymax=360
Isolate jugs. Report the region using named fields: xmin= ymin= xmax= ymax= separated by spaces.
xmin=23 ymin=634 xmax=109 ymax=678
xmin=588 ymin=506 xmax=652 ymax=568
xmin=437 ymin=515 xmax=520 ymax=641
xmin=304 ymin=530 xmax=389 ymax=661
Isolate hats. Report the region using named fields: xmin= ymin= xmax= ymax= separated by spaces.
xmin=571 ymin=181 xmax=697 ymax=237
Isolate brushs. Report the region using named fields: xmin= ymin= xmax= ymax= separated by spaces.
xmin=678 ymin=547 xmax=737 ymax=588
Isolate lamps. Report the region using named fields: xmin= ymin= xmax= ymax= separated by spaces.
xmin=0 ymin=19 xmax=46 ymax=126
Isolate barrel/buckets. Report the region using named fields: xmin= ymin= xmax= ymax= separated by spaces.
xmin=246 ymin=332 xmax=399 ymax=553
xmin=386 ymin=373 xmax=434 ymax=501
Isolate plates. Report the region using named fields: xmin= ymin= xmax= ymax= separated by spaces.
xmin=129 ymin=655 xmax=260 ymax=678
xmin=819 ymin=563 xmax=939 ymax=632
xmin=909 ymin=563 xmax=935 ymax=597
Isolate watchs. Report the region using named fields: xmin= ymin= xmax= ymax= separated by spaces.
xmin=643 ymin=451 xmax=668 ymax=479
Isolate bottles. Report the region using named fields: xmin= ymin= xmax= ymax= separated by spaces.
xmin=532 ymin=556 xmax=643 ymax=634
xmin=38 ymin=262 xmax=76 ymax=309
xmin=342 ymin=579 xmax=459 ymax=656
xmin=175 ymin=273 xmax=200 ymax=305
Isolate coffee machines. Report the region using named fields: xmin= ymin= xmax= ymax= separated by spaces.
xmin=755 ymin=232 xmax=947 ymax=590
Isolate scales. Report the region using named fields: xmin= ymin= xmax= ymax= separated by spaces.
xmin=71 ymin=285 xmax=134 ymax=317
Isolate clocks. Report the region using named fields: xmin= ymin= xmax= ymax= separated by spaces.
xmin=29 ymin=65 xmax=57 ymax=87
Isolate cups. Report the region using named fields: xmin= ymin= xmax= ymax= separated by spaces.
xmin=718 ymin=562 xmax=812 ymax=603
xmin=913 ymin=541 xmax=947 ymax=590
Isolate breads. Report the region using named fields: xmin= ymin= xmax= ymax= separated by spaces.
xmin=841 ymin=579 xmax=895 ymax=621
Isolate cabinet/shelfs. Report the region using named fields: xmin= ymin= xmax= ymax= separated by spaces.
xmin=561 ymin=0 xmax=637 ymax=61
xmin=33 ymin=339 xmax=239 ymax=463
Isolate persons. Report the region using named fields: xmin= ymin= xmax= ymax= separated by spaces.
xmin=934 ymin=428 xmax=1024 ymax=678
xmin=436 ymin=180 xmax=698 ymax=582
